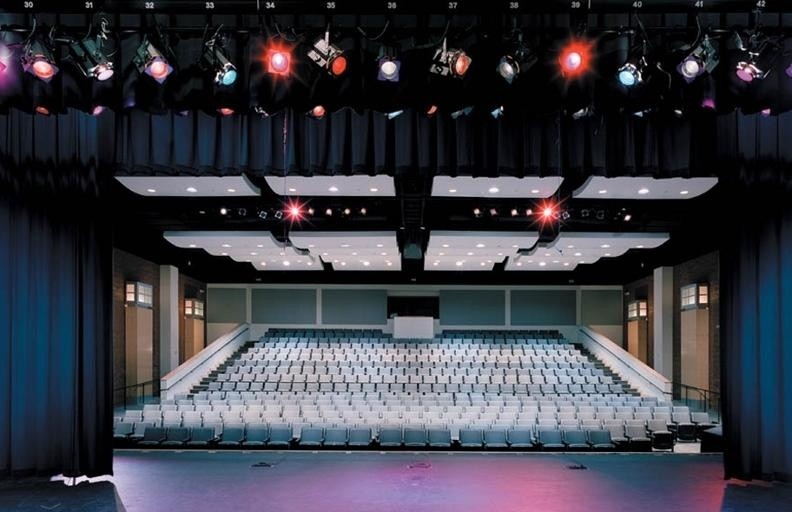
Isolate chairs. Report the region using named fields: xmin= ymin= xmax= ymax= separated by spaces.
xmin=113 ymin=328 xmax=717 ymax=447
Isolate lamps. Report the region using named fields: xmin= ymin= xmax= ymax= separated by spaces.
xmin=0 ymin=0 xmax=792 ymax=120
